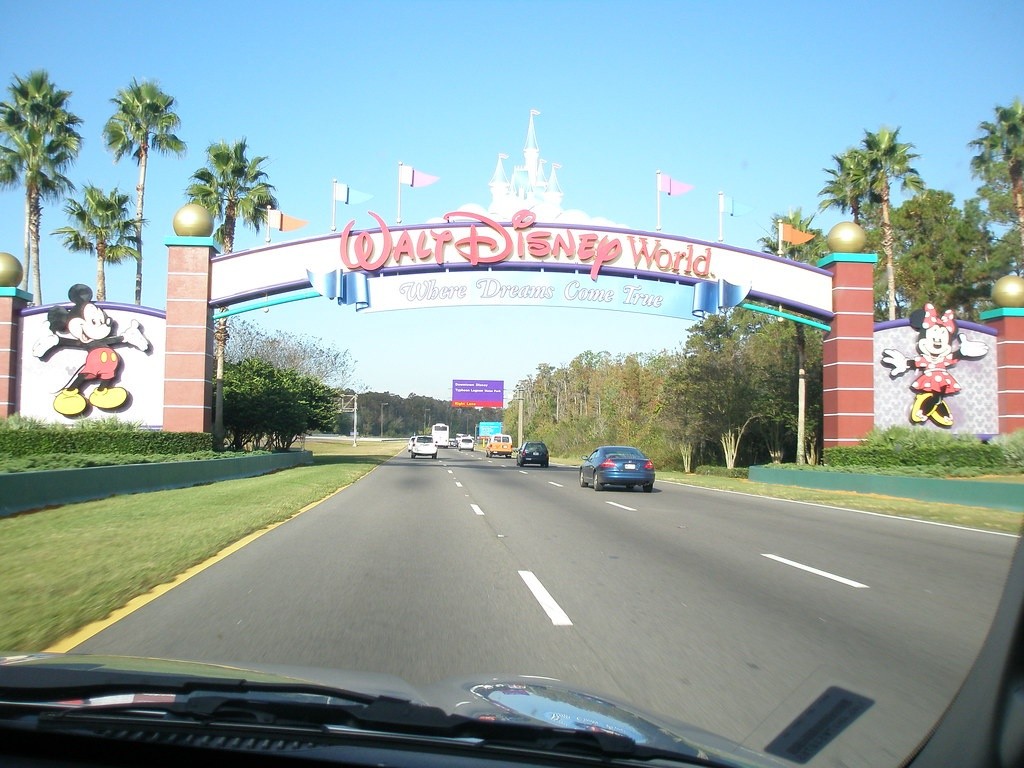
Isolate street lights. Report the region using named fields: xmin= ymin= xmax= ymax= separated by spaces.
xmin=380 ymin=402 xmax=389 ymax=438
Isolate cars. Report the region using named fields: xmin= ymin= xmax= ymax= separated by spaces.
xmin=407 ymin=435 xmax=438 ymax=459
xmin=449 ymin=433 xmax=476 ymax=450
xmin=517 ymin=441 xmax=550 ymax=468
xmin=486 ymin=434 xmax=513 ymax=459
xmin=579 ymin=445 xmax=655 ymax=492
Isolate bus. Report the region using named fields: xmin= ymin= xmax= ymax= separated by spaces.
xmin=431 ymin=422 xmax=450 ymax=448
